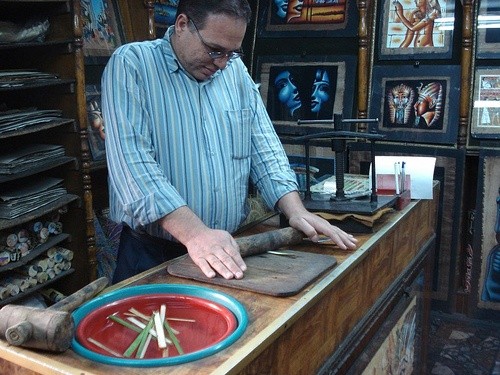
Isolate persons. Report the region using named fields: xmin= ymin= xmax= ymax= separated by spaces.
xmin=101 ymin=0 xmax=358 ymax=287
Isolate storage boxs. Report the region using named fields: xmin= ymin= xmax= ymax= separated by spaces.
xmin=375 ymin=173 xmax=411 ymax=212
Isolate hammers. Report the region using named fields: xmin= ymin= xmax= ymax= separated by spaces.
xmin=0 ymin=276 xmax=109 ymax=354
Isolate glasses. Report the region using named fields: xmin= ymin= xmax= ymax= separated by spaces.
xmin=188 ymin=16 xmax=244 ymax=62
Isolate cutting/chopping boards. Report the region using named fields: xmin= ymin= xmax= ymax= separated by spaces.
xmin=167 ymin=250 xmax=337 ymax=295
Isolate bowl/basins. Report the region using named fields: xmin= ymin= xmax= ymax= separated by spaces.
xmin=71 ymin=284 xmax=247 ymax=367
xmin=75 ymin=294 xmax=237 ymax=359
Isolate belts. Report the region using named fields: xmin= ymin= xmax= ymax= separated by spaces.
xmin=123 ymin=225 xmax=187 ymax=252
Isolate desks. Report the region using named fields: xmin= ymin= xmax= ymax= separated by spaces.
xmin=0 ymin=173 xmax=441 ymax=375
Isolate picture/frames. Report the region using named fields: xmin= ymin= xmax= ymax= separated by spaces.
xmin=66 ymin=0 xmax=499 ymax=321
xmin=77 ymin=0 xmax=499 ymax=320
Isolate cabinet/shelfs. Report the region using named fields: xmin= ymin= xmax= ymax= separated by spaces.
xmin=0 ymin=0 xmax=97 ymax=310
